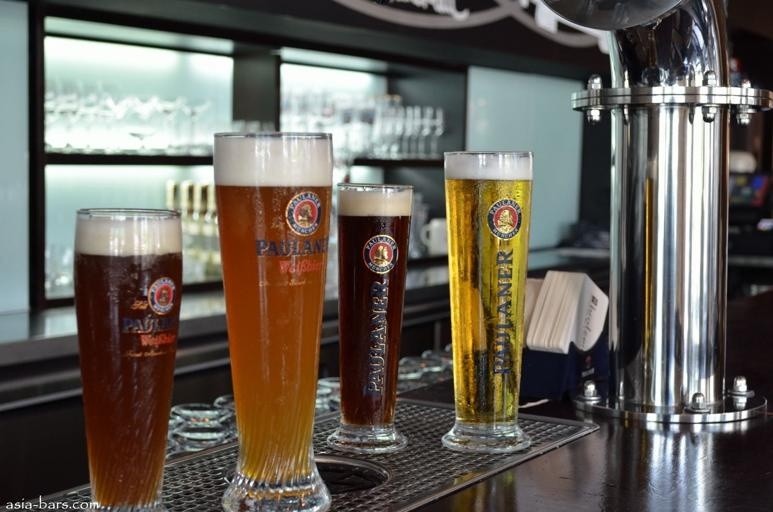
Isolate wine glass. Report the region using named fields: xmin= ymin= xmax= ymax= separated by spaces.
xmin=281 ymin=91 xmax=446 ymax=159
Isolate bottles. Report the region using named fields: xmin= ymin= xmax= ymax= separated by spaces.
xmin=161 ymin=180 xmax=219 ymax=281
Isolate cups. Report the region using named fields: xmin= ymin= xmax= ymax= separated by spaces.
xmin=326 ymin=182 xmax=413 ymax=456
xmin=43 ymin=88 xmax=208 ymax=156
xmin=70 ymin=206 xmax=184 ymax=512
xmin=44 ymin=241 xmax=71 ymax=288
xmin=421 ymin=219 xmax=447 ymax=255
xmin=211 ymin=131 xmax=336 ymax=512
xmin=437 ymin=148 xmax=536 ymax=453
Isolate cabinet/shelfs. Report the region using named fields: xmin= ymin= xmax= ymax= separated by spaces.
xmin=274 ymin=32 xmax=468 ymax=273
xmin=26 ymin=1 xmax=237 ymax=318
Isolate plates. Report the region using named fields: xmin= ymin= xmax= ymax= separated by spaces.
xmin=152 ymin=343 xmax=454 ymax=456
